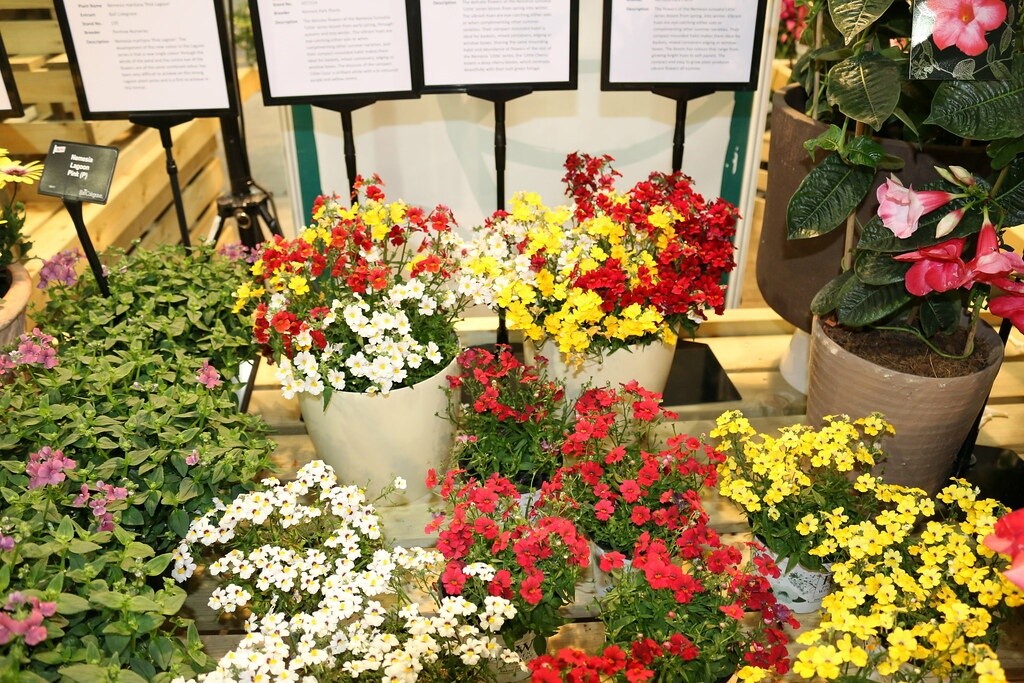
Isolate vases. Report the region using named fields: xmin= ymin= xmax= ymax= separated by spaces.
xmin=806 ymin=311 xmax=1005 ymax=503
xmin=747 ymin=518 xmax=836 ymax=614
xmin=465 ymin=617 xmax=546 ymax=682
xmin=590 ymin=537 xmax=680 ymax=600
xmin=469 ymin=490 xmax=549 ymax=533
xmin=868 ymin=635 xmax=960 ymax=682
xmin=754 ymin=83 xmax=983 ymax=336
xmin=605 ymin=622 xmax=741 ymax=683
xmin=297 ymin=334 xmax=462 ymax=507
xmin=525 ymin=321 xmax=680 ymax=458
xmin=1 ymin=260 xmax=33 ymax=349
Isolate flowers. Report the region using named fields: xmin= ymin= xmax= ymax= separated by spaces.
xmin=0 ymin=0 xmax=1024 ymax=683
